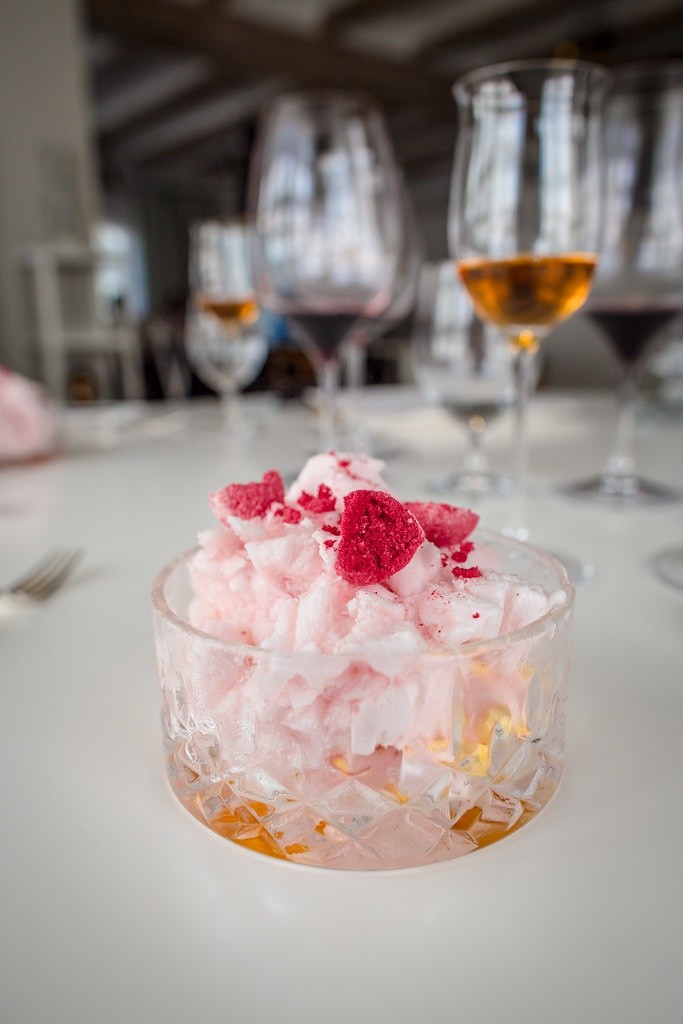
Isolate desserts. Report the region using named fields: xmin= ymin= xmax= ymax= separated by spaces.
xmin=185 ymin=452 xmax=568 ymax=798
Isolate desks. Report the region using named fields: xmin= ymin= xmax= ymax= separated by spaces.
xmin=1 ymin=381 xmax=681 ymax=1024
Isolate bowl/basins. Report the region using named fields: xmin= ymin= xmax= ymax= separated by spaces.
xmin=152 ymin=526 xmax=577 ymax=874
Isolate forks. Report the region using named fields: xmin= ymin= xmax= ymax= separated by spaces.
xmin=0 ymin=548 xmax=81 ymax=606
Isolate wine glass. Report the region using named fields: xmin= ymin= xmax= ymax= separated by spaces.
xmin=181 ymin=57 xmax=683 ymax=510
